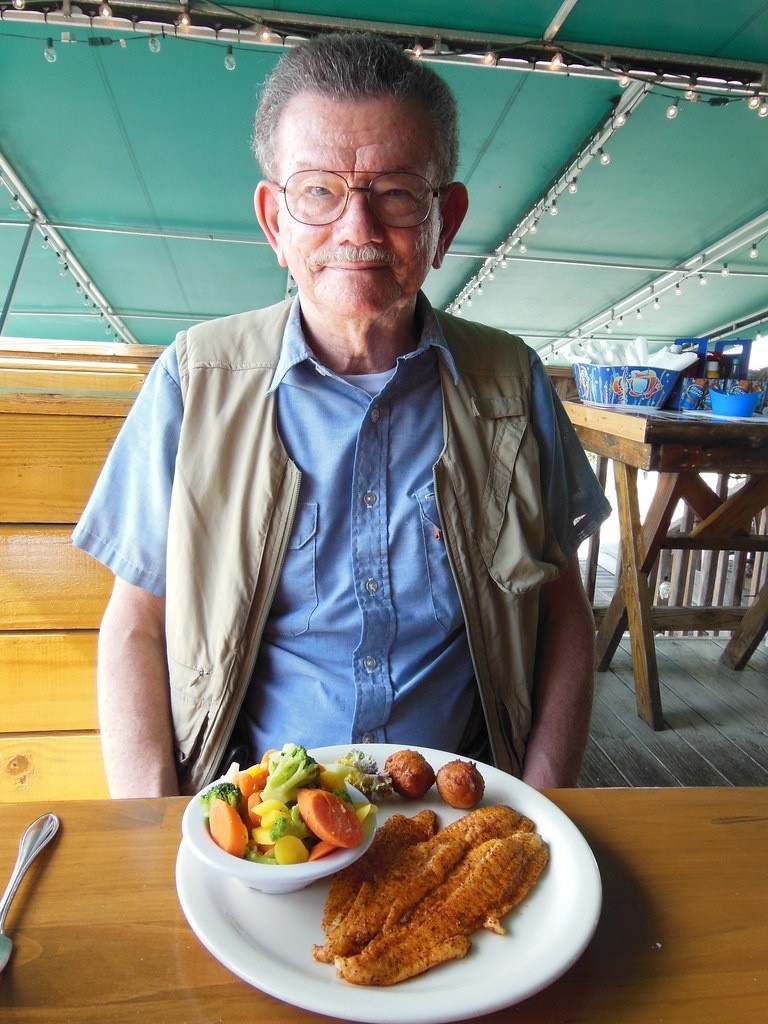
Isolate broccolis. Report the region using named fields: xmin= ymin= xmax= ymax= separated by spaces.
xmin=202 ymin=783 xmax=242 ymax=818
xmin=246 ymin=852 xmax=277 ymax=866
xmin=334 ymin=789 xmax=351 ymax=802
xmin=271 ymin=805 xmax=313 ymax=842
xmin=260 ymin=743 xmax=320 ymax=804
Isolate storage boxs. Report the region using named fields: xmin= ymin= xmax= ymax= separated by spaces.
xmin=572 ymin=362 xmax=680 ymax=410
xmin=725 ymin=379 xmax=768 ymax=413
xmin=666 ymin=378 xmax=725 ymax=410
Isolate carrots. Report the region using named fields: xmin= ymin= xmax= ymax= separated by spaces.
xmin=208 ymin=765 xmax=364 ymax=860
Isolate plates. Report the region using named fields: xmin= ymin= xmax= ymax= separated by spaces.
xmin=175 ymin=743 xmax=603 ymax=1024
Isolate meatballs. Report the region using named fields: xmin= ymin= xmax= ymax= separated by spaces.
xmin=436 ymin=759 xmax=485 ymax=808
xmin=385 ymin=749 xmax=435 ymax=797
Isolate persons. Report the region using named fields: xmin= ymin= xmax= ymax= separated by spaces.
xmin=66 ymin=31 xmax=613 ymax=800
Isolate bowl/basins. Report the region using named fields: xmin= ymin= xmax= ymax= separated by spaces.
xmin=708 ymin=386 xmax=765 ymax=418
xmin=179 ymin=769 xmax=377 ymax=894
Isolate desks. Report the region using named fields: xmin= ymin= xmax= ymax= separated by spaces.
xmin=561 ymin=401 xmax=768 ymax=733
xmin=0 ymin=787 xmax=768 ymax=1023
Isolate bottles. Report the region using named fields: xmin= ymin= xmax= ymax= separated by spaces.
xmin=705 ymin=358 xmax=741 ymax=379
xmin=680 ymin=378 xmax=724 ymax=409
xmin=727 ymin=380 xmax=767 ymax=415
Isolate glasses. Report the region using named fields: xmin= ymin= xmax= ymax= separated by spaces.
xmin=277 ymin=169 xmax=442 ymax=228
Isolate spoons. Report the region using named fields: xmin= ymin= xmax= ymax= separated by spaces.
xmin=0 ymin=811 xmax=61 ymax=974
